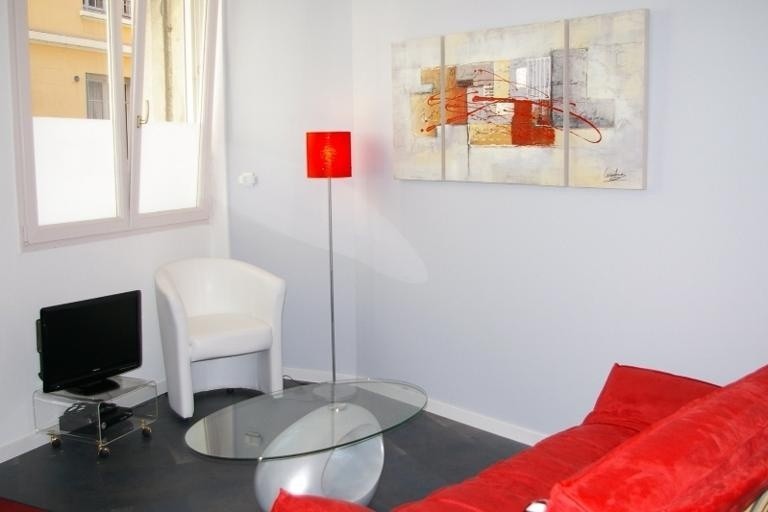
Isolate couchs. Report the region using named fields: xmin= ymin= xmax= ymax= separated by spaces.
xmin=269 ymin=362 xmax=723 ymax=511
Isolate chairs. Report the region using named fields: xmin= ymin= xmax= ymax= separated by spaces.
xmin=155 ymin=257 xmax=286 ymax=420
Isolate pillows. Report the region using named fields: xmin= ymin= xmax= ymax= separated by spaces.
xmin=541 ymin=364 xmax=767 ymax=510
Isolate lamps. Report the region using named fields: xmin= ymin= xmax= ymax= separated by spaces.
xmin=306 ymin=132 xmax=357 ymax=405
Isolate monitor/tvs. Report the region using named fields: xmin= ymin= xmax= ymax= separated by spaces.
xmin=36 ymin=289 xmax=142 ymax=396
